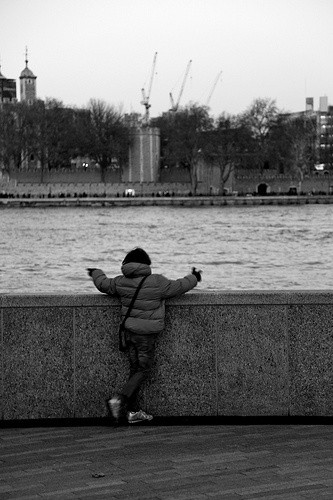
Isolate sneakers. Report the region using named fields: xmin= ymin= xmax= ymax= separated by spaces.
xmin=106 ymin=394 xmax=124 ymax=422
xmin=127 ymin=409 xmax=153 ymax=424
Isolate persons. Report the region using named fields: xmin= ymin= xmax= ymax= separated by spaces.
xmin=84 ymin=246 xmax=202 ymax=426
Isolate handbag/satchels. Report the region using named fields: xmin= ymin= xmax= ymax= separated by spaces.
xmin=118 ymin=324 xmax=129 ymax=352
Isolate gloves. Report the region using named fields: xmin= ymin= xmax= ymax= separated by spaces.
xmin=86 ymin=268 xmax=98 ymax=276
xmin=192 ymin=267 xmax=202 ymax=281
